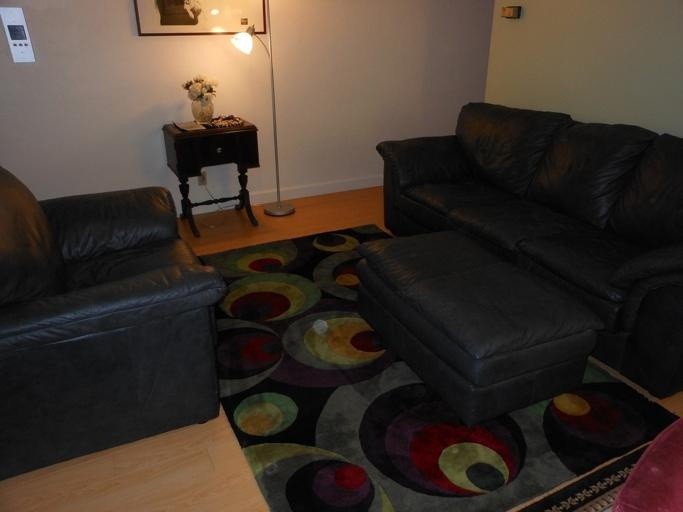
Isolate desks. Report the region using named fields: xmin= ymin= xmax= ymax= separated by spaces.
xmin=164 ymin=117 xmax=261 ymax=237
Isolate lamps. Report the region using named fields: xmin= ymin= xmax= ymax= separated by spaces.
xmin=233 ymin=27 xmax=295 ymax=217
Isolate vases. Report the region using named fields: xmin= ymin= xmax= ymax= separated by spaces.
xmin=191 ymin=97 xmax=215 ymax=121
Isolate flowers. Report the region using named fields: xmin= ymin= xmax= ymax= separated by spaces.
xmin=182 ymin=74 xmax=220 ymax=100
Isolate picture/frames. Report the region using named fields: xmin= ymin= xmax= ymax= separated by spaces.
xmin=133 ymin=0 xmax=266 ymax=35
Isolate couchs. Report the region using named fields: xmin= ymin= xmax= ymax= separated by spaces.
xmin=374 ymin=101 xmax=683 ymax=330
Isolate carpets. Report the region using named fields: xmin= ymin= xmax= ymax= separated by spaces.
xmin=198 ymin=223 xmax=683 ymax=512
xmin=501 ymin=440 xmax=649 ymax=512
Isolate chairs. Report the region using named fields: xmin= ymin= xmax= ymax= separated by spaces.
xmin=0 ymin=165 xmax=228 ymax=480
